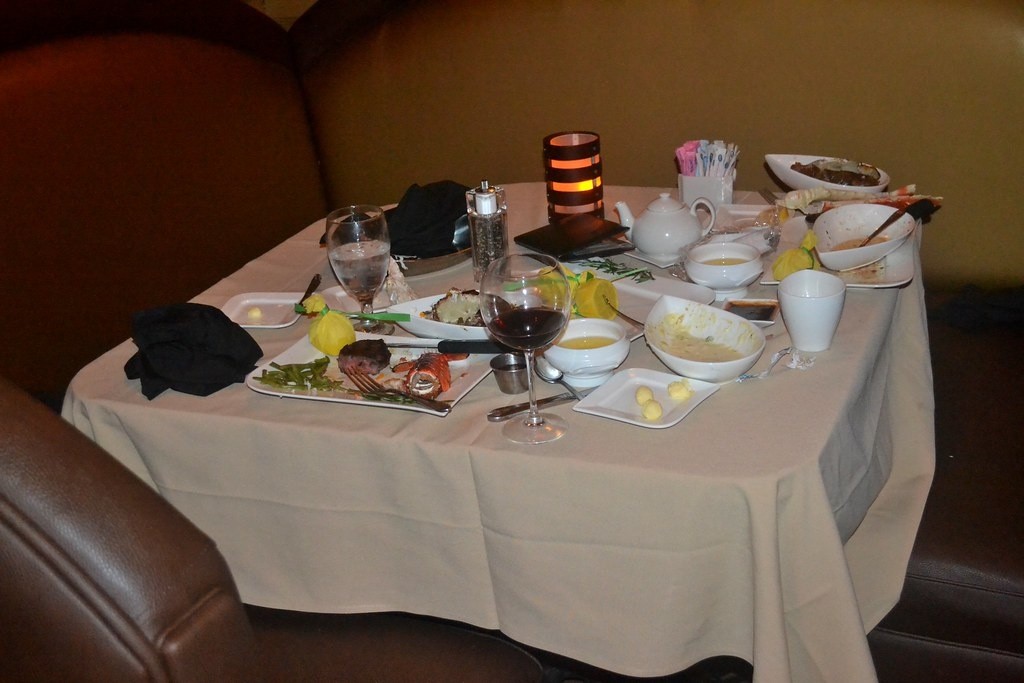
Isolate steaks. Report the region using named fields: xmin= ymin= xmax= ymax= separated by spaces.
xmin=338 ymin=339 xmax=391 ymax=375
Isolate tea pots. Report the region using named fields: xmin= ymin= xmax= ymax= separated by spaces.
xmin=615 ymin=192 xmax=716 ymax=261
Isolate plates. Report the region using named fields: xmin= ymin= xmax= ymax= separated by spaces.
xmin=717 ymin=203 xmax=777 ymax=229
xmin=760 ymin=215 xmax=915 ymax=288
xmin=608 ymin=272 xmax=716 ymax=342
xmin=220 ymin=291 xmax=305 ymax=328
xmin=400 ymin=250 xmax=472 ymax=277
xmin=247 ymin=330 xmax=500 ymax=417
xmin=318 ymin=285 xmax=393 ymax=311
xmin=572 ymin=368 xmax=720 ymax=428
xmin=623 ymin=250 xmax=681 ymax=268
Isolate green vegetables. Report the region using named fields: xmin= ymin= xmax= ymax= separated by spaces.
xmin=252 ymin=355 xmax=417 ymax=405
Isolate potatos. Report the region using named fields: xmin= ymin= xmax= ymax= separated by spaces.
xmin=791 ymin=157 xmax=882 ymax=186
xmin=432 ymin=289 xmax=512 ymax=327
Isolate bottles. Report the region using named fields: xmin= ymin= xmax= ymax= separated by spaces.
xmin=467 ymin=179 xmax=512 ymax=283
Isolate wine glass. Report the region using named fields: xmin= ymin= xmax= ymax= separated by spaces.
xmin=478 ymin=252 xmax=571 ymax=442
xmin=325 ymin=203 xmax=395 ymax=334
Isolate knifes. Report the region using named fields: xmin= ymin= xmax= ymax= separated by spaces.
xmin=385 ymin=338 xmax=500 ymax=354
xmin=487 ymin=385 xmax=600 ymax=420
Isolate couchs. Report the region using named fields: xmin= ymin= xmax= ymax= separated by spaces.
xmin=0 ymin=0 xmax=1023 ymax=683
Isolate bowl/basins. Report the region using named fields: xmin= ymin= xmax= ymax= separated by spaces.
xmin=542 ymin=317 xmax=630 ymax=387
xmin=645 ymin=294 xmax=766 ymax=385
xmin=765 ymin=154 xmax=890 ymax=201
xmin=385 ymin=293 xmax=542 ymax=339
xmin=721 ymin=297 xmax=779 ymax=329
xmin=683 ymin=242 xmax=763 ymax=301
xmin=811 ymin=203 xmax=915 ymax=272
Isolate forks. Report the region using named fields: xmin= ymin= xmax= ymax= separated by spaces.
xmin=343 ymin=365 xmax=452 ymax=412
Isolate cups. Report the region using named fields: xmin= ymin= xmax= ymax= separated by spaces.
xmin=777 ymin=269 xmax=846 ymax=352
xmin=490 ymin=352 xmax=529 ymax=394
xmin=679 ymin=169 xmax=738 ymax=206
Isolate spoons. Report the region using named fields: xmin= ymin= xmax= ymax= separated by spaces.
xmin=533 ymin=355 xmax=585 ymax=402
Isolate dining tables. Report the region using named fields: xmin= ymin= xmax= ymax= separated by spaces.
xmin=63 ymin=186 xmax=939 ymax=683
